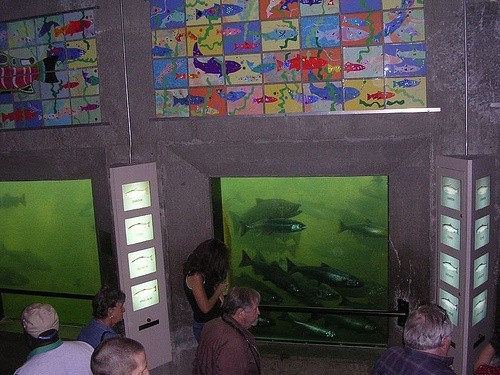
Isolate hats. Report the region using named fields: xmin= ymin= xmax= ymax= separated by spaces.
xmin=20 ymin=303 xmax=59 ymax=339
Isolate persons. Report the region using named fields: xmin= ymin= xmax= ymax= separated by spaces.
xmin=13 ymin=301 xmax=94 ymax=375
xmin=76 ymin=285 xmax=126 ymax=349
xmin=90 ymin=336 xmax=149 ymax=375
xmin=369 ymin=304 xmax=457 ymax=375
xmin=182 ymin=238 xmax=232 ymax=344
xmin=194 ymin=286 xmax=261 ymax=375
xmin=474 ymin=333 xmax=500 ymax=373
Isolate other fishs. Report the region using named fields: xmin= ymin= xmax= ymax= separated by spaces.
xmin=0 ymin=193 xmax=27 ymax=208
xmin=0 ymin=245 xmax=53 ymax=271
xmin=221 ymin=181 xmax=388 ymax=338
xmin=0 ymin=268 xmax=30 ymax=286
